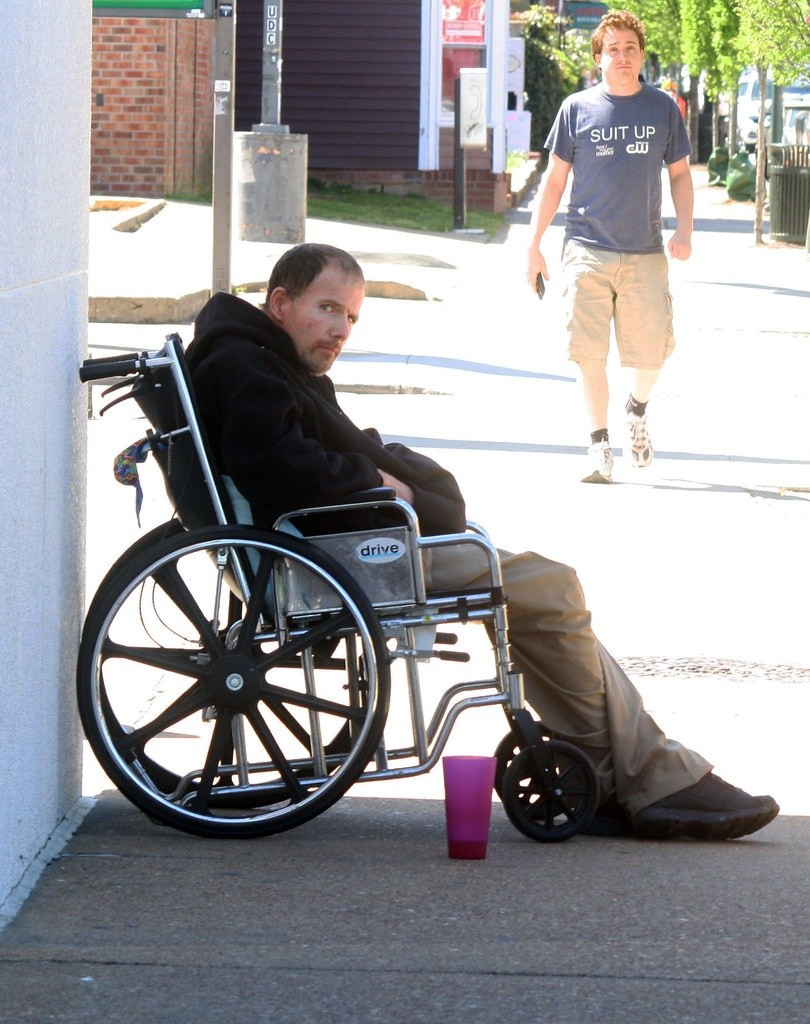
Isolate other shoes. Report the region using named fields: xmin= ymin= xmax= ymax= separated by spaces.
xmin=567 ymin=802 xmax=633 ymax=836
xmin=635 ymin=771 xmax=779 ymax=841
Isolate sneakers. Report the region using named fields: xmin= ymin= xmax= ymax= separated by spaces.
xmin=581 ymin=434 xmax=613 ymax=484
xmin=621 ymin=407 xmax=654 ymax=469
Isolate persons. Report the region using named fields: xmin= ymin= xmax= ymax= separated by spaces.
xmin=524 ymin=9 xmax=694 ymax=486
xmin=177 ymin=244 xmax=779 ymax=842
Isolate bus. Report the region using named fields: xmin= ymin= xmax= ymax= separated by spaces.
xmin=737 ymin=70 xmax=810 ymax=152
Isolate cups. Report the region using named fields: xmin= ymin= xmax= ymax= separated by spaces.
xmin=442 ymin=755 xmax=497 ymax=860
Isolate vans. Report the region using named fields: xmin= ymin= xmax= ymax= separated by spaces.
xmin=751 ymin=100 xmax=810 ymax=179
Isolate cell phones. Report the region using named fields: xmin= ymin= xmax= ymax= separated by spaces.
xmin=537 ymin=273 xmax=545 ymax=300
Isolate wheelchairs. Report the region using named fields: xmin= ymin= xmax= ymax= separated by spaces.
xmin=75 ymin=334 xmax=600 ymax=844
